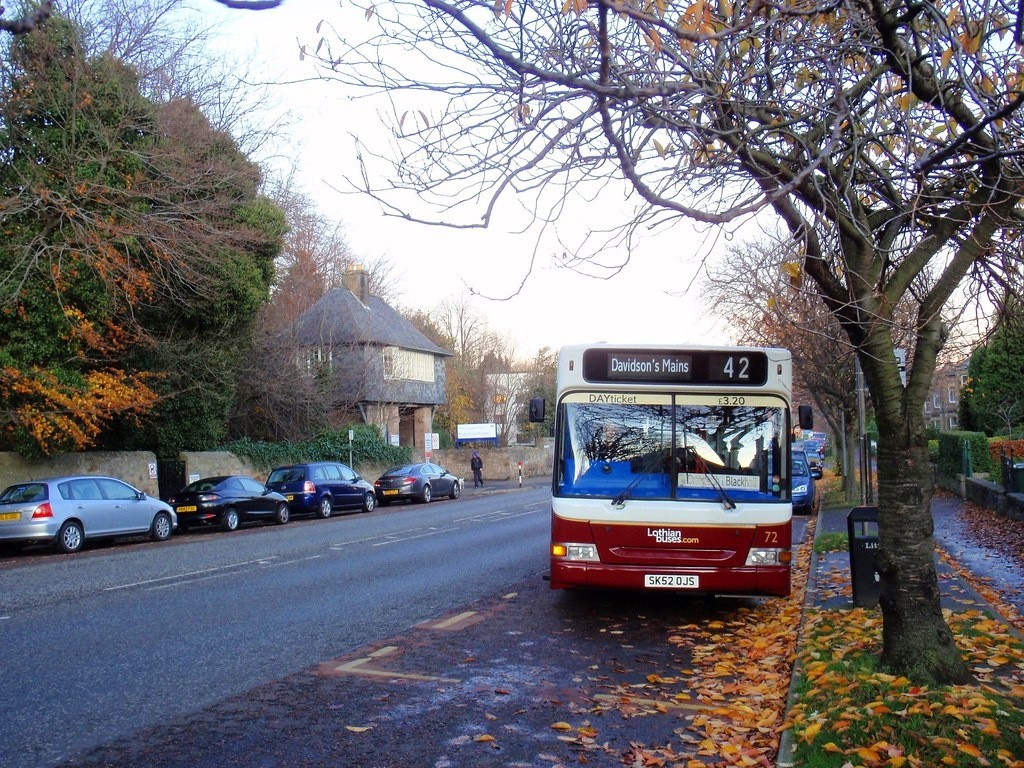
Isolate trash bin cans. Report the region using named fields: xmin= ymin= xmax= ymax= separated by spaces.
xmin=846 ymin=505 xmax=881 ymax=607
xmin=1012 ymin=462 xmax=1024 ymax=493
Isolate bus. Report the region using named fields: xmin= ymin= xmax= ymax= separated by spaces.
xmin=528 ymin=341 xmax=814 ymax=599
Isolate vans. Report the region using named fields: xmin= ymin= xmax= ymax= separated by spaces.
xmin=0 ymin=475 xmax=178 ymax=553
xmin=265 ymin=461 xmax=376 ymax=519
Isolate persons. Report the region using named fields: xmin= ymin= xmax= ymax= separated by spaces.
xmin=471 ymin=453 xmax=483 ymax=488
xmin=597 ymin=423 xmax=641 ymax=472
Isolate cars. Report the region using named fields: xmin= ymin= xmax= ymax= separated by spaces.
xmin=766 ymin=447 xmax=823 ymax=514
xmin=799 ymin=431 xmax=832 ymax=481
xmin=169 ymin=475 xmax=290 ymax=533
xmin=374 ymin=463 xmax=460 ymax=505
